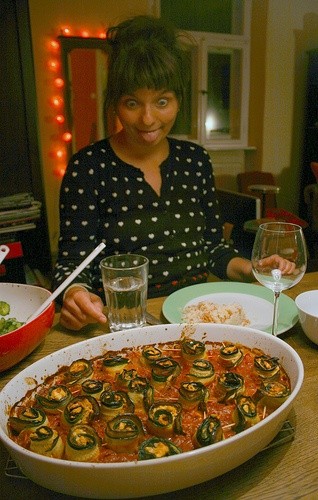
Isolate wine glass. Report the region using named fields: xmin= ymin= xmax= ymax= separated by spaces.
xmin=251 ymin=222 xmax=308 ymax=337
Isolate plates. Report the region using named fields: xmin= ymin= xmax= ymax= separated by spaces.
xmin=161 ymin=281 xmax=300 ymax=336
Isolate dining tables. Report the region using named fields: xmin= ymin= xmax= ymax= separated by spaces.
xmin=0 ymin=271 xmax=318 ymax=500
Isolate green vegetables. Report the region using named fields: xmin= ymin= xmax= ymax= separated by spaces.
xmin=0 ymin=301 xmax=25 ymax=336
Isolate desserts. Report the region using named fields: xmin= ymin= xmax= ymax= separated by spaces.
xmin=195 ymin=301 xmax=245 ymax=326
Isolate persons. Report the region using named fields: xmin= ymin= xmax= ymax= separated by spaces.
xmin=54 ymin=15 xmax=296 ymax=332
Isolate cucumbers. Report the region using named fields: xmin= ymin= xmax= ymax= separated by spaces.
xmin=9 ymin=340 xmax=290 ymax=461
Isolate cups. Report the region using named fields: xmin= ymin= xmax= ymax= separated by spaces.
xmin=100 ymin=254 xmax=149 ymax=333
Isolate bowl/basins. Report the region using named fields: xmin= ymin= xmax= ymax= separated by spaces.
xmin=295 ymin=290 xmax=318 ymax=346
xmin=0 ymin=283 xmax=54 ymax=373
xmin=0 ymin=323 xmax=305 ymax=499
